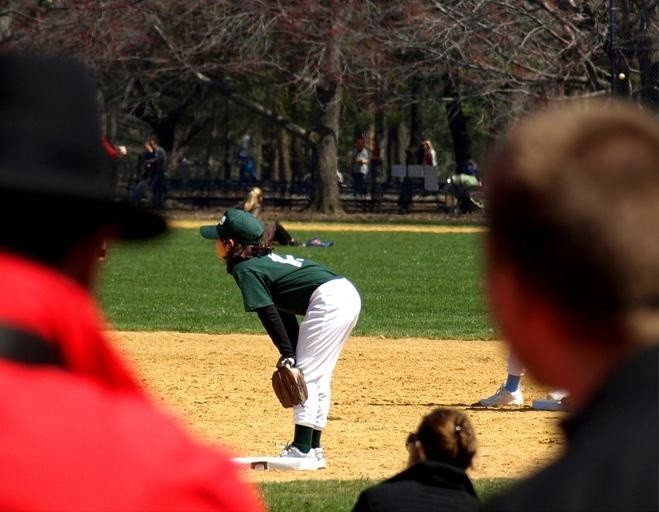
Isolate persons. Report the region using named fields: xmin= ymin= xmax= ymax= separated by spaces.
xmin=474 ymin=97 xmax=658 ymax=511
xmin=478 ymin=344 xmax=572 ymax=412
xmin=198 ymin=208 xmax=362 ymax=471
xmin=99 ymin=134 xmax=297 ymax=247
xmin=1 ymin=54 xmax=270 ymax=510
xmin=352 ymin=408 xmax=485 ymax=512
xmin=350 ymin=138 xmax=480 ymax=200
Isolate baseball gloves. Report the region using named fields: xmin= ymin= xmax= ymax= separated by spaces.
xmin=271 ymin=362 xmax=308 ymax=408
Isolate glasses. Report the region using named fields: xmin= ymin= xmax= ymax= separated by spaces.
xmin=406 ymin=434 xmax=420 ymax=449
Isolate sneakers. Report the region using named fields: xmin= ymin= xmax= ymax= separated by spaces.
xmin=277 ymin=448 xmax=325 ymax=470
xmin=548 ymin=389 xmax=568 ymax=400
xmin=480 ymin=386 xmax=523 ymax=407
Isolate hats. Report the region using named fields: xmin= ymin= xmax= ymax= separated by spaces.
xmin=1 ymin=56 xmax=167 ymax=243
xmin=200 ymin=208 xmax=264 ymax=244
